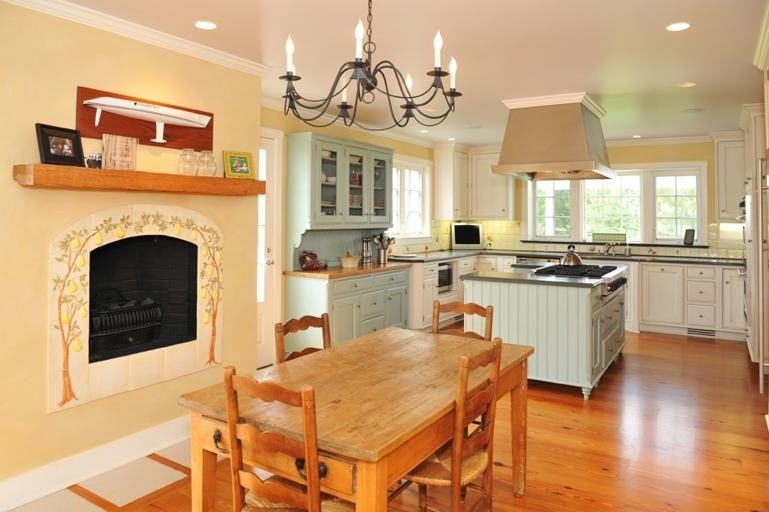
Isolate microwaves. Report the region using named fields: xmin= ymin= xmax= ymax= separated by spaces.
xmin=438 ymin=266 xmax=453 ymax=293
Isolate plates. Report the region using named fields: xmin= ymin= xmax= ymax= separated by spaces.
xmin=392 ymin=254 xmax=417 ymax=258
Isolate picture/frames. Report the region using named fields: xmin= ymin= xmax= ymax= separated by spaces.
xmin=222 ymin=149 xmax=255 ymax=180
xmin=35 ymin=123 xmax=86 ymax=166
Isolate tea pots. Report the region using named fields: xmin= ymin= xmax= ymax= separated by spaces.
xmin=559 ymin=244 xmax=583 ymax=266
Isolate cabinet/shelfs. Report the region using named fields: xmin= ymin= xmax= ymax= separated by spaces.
xmin=412 ymin=263 xmax=438 ymax=329
xmin=283 ymin=268 xmax=410 ymax=354
xmin=455 ymin=253 xmax=516 ymax=286
xmin=285 ymin=131 xmax=395 ymax=231
xmin=715 ymin=138 xmax=745 ymax=223
xmin=638 ymin=263 xmax=686 ymax=330
xmin=723 ymin=264 xmax=747 ymax=332
xmin=685 ymin=264 xmax=721 ymax=331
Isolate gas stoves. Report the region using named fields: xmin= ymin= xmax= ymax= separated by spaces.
xmin=535 ymin=264 xmax=617 ymax=278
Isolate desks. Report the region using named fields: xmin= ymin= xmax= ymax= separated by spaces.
xmin=178 ymin=326 xmax=535 ymax=511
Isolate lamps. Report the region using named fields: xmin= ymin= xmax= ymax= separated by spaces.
xmin=279 ymin=1 xmax=462 ymax=134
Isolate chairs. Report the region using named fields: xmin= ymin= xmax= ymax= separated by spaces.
xmin=223 ymin=366 xmax=355 ymax=511
xmin=274 ymin=312 xmax=331 ymax=366
xmin=400 ymin=337 xmax=502 ymax=512
xmin=432 ymin=300 xmax=493 ymax=342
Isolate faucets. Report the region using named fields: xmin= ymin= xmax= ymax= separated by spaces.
xmin=612 ymin=241 xmax=620 ymax=255
xmin=603 ymin=241 xmax=615 ymax=255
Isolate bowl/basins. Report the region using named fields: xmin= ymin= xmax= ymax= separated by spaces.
xmin=321 ymin=151 xmax=384 ymax=207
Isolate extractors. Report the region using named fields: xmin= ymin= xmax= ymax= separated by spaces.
xmin=491 ymin=103 xmax=618 ymax=180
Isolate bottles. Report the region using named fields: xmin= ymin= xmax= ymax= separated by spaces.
xmin=178 ymin=148 xmax=198 ymax=176
xmin=198 ymin=150 xmax=217 ymax=176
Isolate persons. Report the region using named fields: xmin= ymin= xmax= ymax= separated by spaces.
xmin=233 ymin=159 xmax=248 ymax=174
xmin=50 ymin=142 xmax=74 ymax=157
